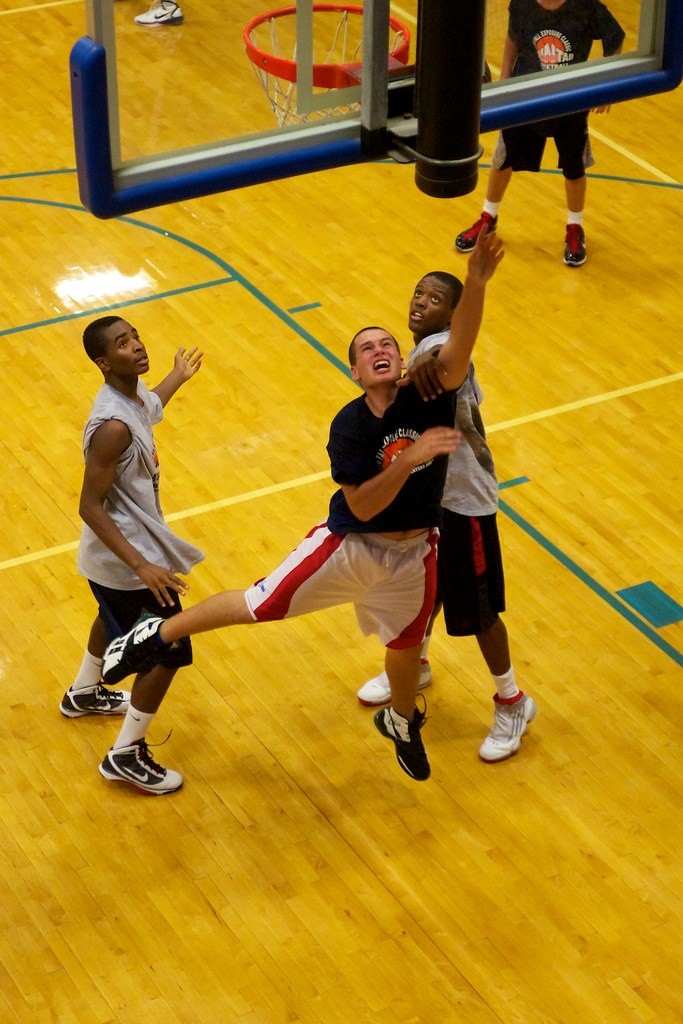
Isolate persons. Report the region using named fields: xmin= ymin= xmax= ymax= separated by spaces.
xmin=100 ymin=222 xmax=539 ymax=781
xmin=59 ymin=315 xmax=204 ymax=797
xmin=454 ymin=1 xmax=626 ymax=268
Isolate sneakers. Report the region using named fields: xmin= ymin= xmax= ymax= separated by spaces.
xmin=374 ymin=693 xmax=431 ymax=781
xmin=357 ymin=658 xmax=432 ymax=706
xmin=59 ymin=680 xmax=132 ymax=717
xmin=479 ymin=690 xmax=536 ymax=762
xmin=454 ymin=212 xmax=498 ymax=252
xmin=563 ymin=223 xmax=587 ymax=266
xmin=101 ymin=611 xmax=174 ymax=686
xmin=98 ymin=729 xmax=183 ymax=795
xmin=134 ymin=0 xmax=184 ymax=27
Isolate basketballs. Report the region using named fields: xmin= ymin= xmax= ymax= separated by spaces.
xmin=481 ymin=59 xmax=493 ymax=85
